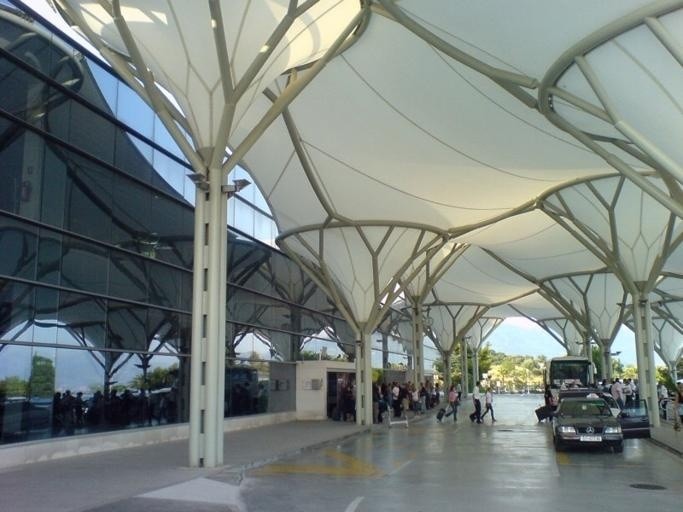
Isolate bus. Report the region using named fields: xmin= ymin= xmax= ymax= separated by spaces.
xmin=540 ymin=355 xmax=599 ymax=407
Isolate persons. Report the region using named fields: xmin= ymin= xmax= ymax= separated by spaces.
xmin=335 ymin=379 xmax=440 ymax=423
xmin=675 ymin=382 xmax=683 ymax=424
xmin=54 ymin=379 xmax=263 ymax=434
xmin=600 ymin=377 xmax=668 ymax=419
xmin=445 ymin=386 xmax=460 ymax=423
xmin=481 ymin=387 xmax=496 ymax=421
xmin=544 ymin=384 xmax=554 ymax=423
xmin=470 ymin=381 xmax=481 ymax=423
xmin=456 ymin=380 xmax=462 ymax=401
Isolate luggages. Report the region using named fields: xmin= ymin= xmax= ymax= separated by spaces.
xmin=437 ymin=405 xmax=450 ymax=420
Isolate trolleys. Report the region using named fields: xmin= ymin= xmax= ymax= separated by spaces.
xmin=384 ymin=405 xmax=409 ymax=428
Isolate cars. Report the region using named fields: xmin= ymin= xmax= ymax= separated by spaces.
xmin=552 ymin=395 xmax=650 ymax=451
xmin=0 ymin=363 xmax=261 ymax=431
xmin=560 ymin=386 xmax=621 ymax=418
